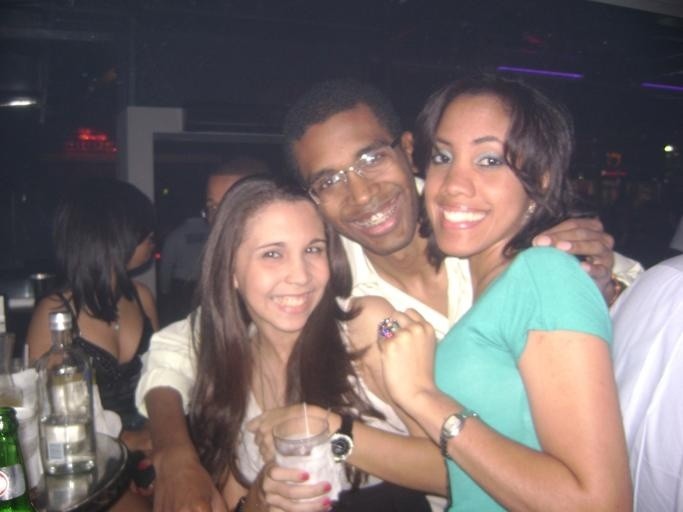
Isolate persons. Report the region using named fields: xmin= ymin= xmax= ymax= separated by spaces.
xmin=191 ymin=172 xmax=449 ymax=511
xmin=203 ymin=154 xmax=269 ymax=220
xmin=133 ymin=74 xmax=643 ymax=512
xmin=26 ymin=174 xmax=160 ymax=512
xmin=157 ymin=217 xmax=210 ymax=321
xmin=603 ymin=250 xmax=682 ymax=512
xmin=381 ymin=69 xmax=632 ymax=512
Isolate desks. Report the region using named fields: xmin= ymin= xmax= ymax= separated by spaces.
xmin=31 ymin=432 xmax=131 ymax=512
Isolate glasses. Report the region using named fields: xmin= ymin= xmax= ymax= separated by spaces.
xmin=301 ymin=137 xmax=398 ymax=205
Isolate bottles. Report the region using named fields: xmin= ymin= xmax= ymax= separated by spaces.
xmin=34 ymin=309 xmax=98 ymax=477
xmin=0 ymin=333 xmax=42 ymax=512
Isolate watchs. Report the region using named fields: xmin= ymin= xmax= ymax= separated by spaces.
xmin=327 ymin=413 xmax=354 ymax=461
xmin=438 ymin=408 xmax=480 ymax=458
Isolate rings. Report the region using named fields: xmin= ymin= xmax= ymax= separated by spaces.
xmin=378 ymin=316 xmax=400 ymax=341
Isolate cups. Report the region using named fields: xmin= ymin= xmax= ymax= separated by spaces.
xmin=271 ymin=416 xmax=338 ymax=503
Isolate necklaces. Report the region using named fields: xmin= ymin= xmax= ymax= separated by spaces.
xmin=105 ymin=306 xmax=126 ymax=332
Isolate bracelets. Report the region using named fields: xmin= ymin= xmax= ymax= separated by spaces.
xmin=605 ymin=276 xmax=624 ymax=307
xmin=231 ymin=495 xmax=247 ymax=510
